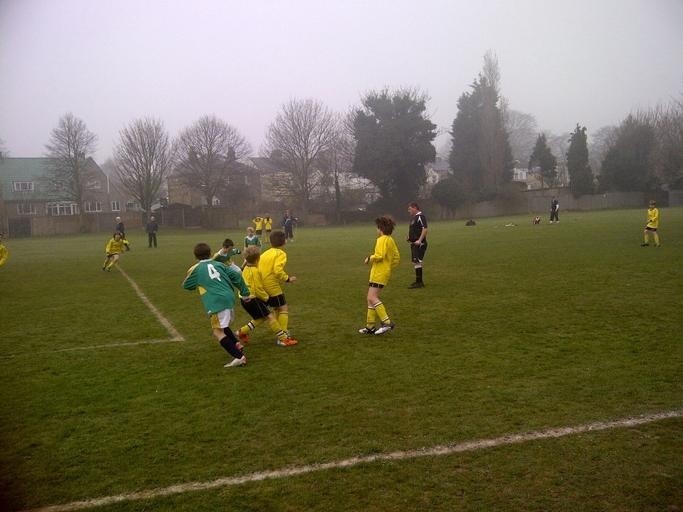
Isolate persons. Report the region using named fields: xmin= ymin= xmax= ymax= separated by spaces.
xmin=114 ymin=217 xmax=130 ymax=251
xmin=182 ymin=208 xmax=298 ymax=368
xmin=0 ymin=234 xmax=8 ymax=265
xmin=407 ymin=202 xmax=428 ymax=289
xmin=102 ymin=233 xmax=131 ymax=272
xmin=549 ymin=195 xmax=560 ymax=224
xmin=640 ymin=200 xmax=660 ymax=247
xmin=358 ymin=216 xmax=401 ymax=334
xmin=145 ymin=216 xmax=159 ymax=248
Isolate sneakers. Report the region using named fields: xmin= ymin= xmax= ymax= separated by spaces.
xmin=407 ymin=282 xmax=424 ymax=288
xmin=223 ymin=328 xmax=298 ymax=368
xmin=358 ymin=326 xmax=376 ymax=334
xmin=374 ymin=319 xmax=394 ymax=335
xmin=640 ymin=243 xmax=648 ymax=247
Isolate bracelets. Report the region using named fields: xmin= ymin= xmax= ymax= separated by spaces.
xmin=368 ymin=255 xmax=370 ymax=262
xmin=650 ymin=220 xmax=652 ymax=222
